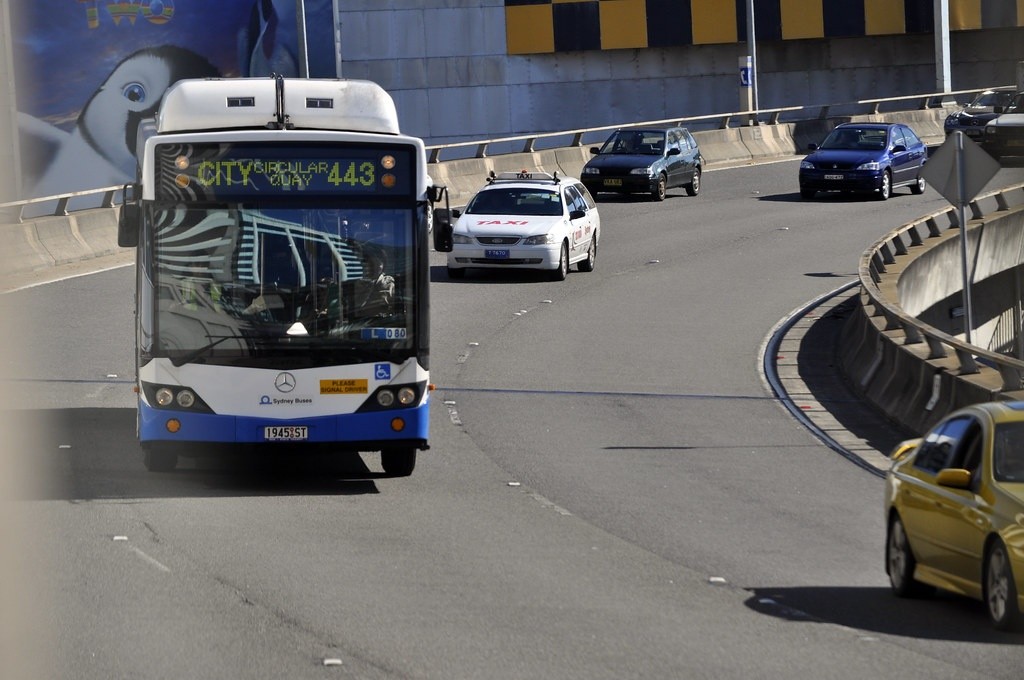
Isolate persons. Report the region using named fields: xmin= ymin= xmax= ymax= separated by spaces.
xmin=347 ymin=245 xmax=396 ymax=327
xmin=176 ymin=251 xmax=222 ymax=312
xmin=620 ymin=135 xmax=637 ymax=151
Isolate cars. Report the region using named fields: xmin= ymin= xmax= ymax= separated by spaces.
xmin=884 ymin=401 xmax=1024 ymax=634
xmin=580 ymin=126 xmax=706 ymax=202
xmin=448 ymin=171 xmax=600 ymax=280
xmin=944 ymin=88 xmax=1019 ymax=143
xmin=799 ymin=122 xmax=927 ymax=200
xmin=983 ymin=91 xmax=1024 ymax=159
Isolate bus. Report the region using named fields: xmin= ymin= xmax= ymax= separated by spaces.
xmin=118 ymin=73 xmax=454 ymax=476
